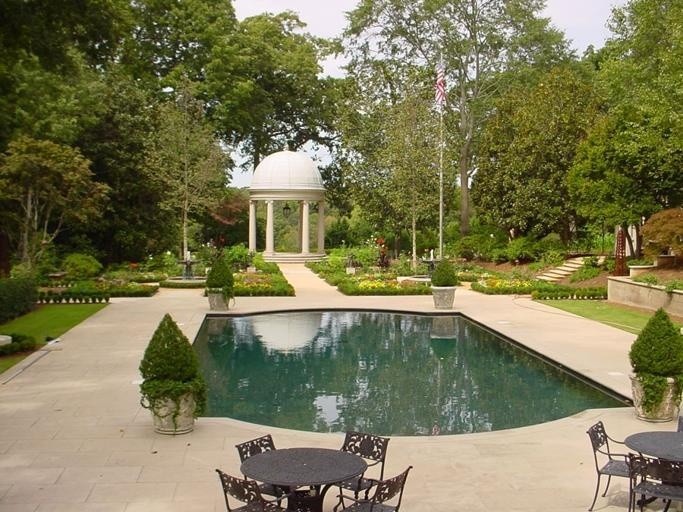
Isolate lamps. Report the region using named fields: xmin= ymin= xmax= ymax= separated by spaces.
xmin=282 ymin=201 xmax=290 ymax=219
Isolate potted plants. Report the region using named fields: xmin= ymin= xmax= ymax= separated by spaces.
xmin=430 ymin=316 xmax=460 ymax=365
xmin=136 ymin=310 xmax=208 ymax=436
xmin=202 ymin=257 xmax=235 ymax=312
xmin=627 ymin=308 xmax=682 ymax=422
xmin=429 ymin=257 xmax=459 ymax=309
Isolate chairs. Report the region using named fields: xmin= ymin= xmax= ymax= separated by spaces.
xmin=586 ymin=409 xmax=682 ymax=511
xmin=217 ymin=425 xmax=413 ymax=511
